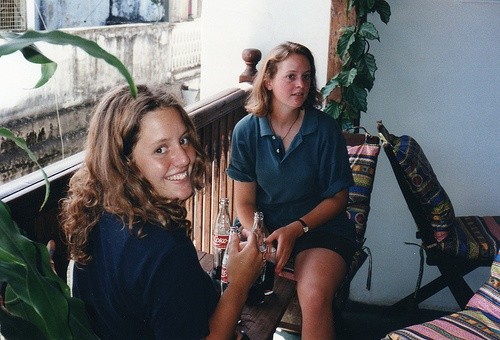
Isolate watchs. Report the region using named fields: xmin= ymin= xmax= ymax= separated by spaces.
xmin=297 ymin=219 xmax=309 ymax=234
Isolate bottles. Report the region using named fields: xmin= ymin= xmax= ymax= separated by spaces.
xmin=247 ymin=211 xmax=267 ymax=306
xmin=212 ymin=197 xmax=231 ymax=282
xmin=220 ymin=226 xmax=241 ymax=296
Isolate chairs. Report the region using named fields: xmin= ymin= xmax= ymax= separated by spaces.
xmin=378 ymin=121 xmax=500 ymax=311
xmin=381 ymin=249 xmax=500 ymax=340
xmin=230 ymin=134 xmax=380 ymax=340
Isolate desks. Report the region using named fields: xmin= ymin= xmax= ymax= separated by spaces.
xmin=198 ymin=251 xmax=297 ymax=340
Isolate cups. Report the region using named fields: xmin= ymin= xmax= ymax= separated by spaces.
xmin=265 ymin=247 xmax=276 ymax=296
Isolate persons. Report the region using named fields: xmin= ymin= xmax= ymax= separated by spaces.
xmin=202 ymin=40 xmax=356 ymax=340
xmin=57 ymin=84 xmax=265 ymax=340
